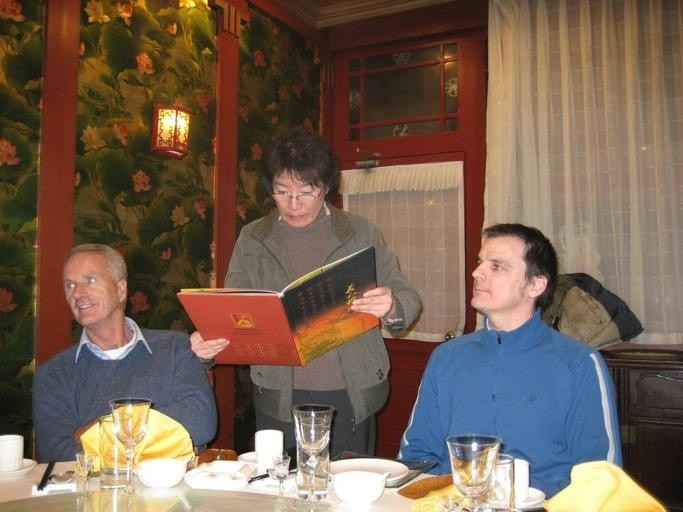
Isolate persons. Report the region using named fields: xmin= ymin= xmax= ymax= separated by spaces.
xmin=30 ymin=242 xmax=220 ymax=465
xmin=185 ymin=126 xmax=422 ymax=465
xmin=394 ymin=221 xmax=625 ymax=512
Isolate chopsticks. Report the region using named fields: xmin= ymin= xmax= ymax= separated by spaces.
xmin=37 ymin=460 xmax=55 ymax=492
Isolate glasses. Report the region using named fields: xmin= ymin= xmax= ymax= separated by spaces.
xmin=272 ymin=184 xmax=327 ymax=205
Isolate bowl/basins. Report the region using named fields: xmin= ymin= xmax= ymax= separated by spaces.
xmin=136 ymin=458 xmax=187 ymax=488
xmin=333 ymin=471 xmax=385 ymax=504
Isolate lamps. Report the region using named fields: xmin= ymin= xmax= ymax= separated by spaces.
xmin=149 ymin=102 xmax=190 ymax=161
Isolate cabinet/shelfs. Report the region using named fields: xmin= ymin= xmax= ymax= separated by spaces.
xmin=606 ymin=355 xmax=682 ymax=512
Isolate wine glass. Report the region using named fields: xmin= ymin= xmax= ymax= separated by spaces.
xmin=290 ymin=404 xmax=337 ymax=512
xmin=270 ymin=451 xmax=290 ymax=498
xmin=106 ymin=395 xmax=153 ymax=485
xmin=445 ymin=432 xmax=502 ymax=512
xmin=72 ymin=451 xmax=98 ymax=500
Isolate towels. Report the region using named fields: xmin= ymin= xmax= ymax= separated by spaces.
xmin=545 ymin=460 xmax=666 ymax=512
xmin=81 ymin=403 xmax=194 ymax=474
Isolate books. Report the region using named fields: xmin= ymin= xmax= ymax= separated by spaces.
xmin=175 ymin=246 xmax=388 ymax=370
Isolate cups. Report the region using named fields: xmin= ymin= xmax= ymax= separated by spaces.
xmin=0 ymin=433 xmax=25 ymax=469
xmin=253 ymin=428 xmax=284 ymax=461
xmin=294 ymin=441 xmax=330 ymax=501
xmin=490 ymin=453 xmax=515 ymax=509
xmin=98 ymin=415 xmax=132 ymax=488
xmin=512 ymin=459 xmax=530 ymax=502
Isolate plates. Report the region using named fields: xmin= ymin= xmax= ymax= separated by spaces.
xmin=330 ymin=450 xmax=437 ymax=488
xmin=326 ymin=457 xmax=408 ymax=480
xmin=514 ymin=486 xmax=546 ymax=508
xmin=237 ymin=452 xmax=261 ymax=465
xmin=0 ymin=456 xmax=37 ymax=483
